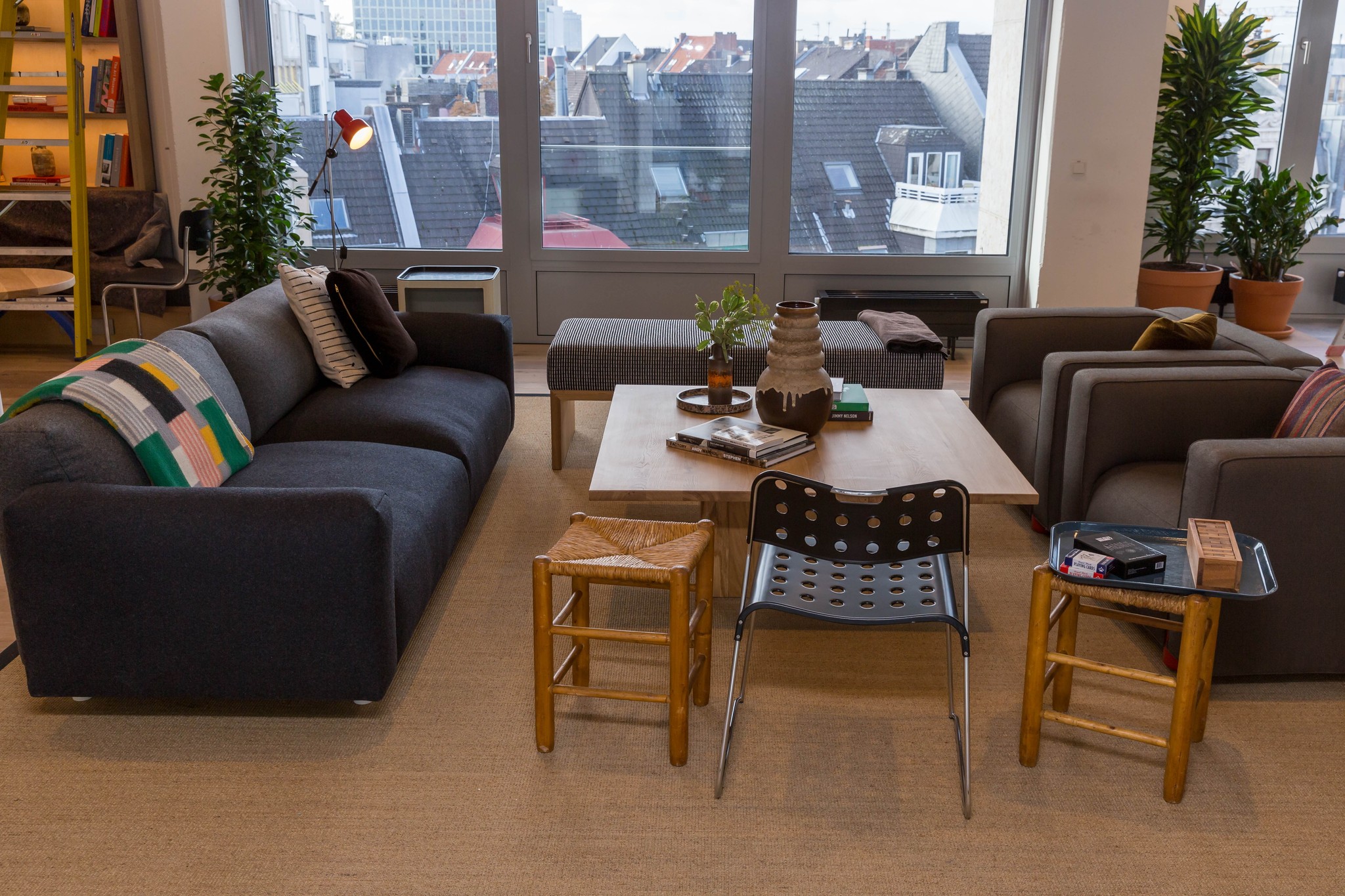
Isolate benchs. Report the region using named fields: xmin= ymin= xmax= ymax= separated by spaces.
xmin=0 ymin=197 xmax=177 ymax=313
xmin=547 ymin=317 xmax=945 ymax=474
xmin=818 ymin=289 xmax=988 ymax=359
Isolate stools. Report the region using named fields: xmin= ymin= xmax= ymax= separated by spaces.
xmin=1017 ymin=556 xmax=1221 ymax=802
xmin=532 ymin=511 xmax=713 ymax=766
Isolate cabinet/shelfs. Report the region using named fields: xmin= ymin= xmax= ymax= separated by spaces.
xmin=2 ymin=0 xmax=156 ymax=196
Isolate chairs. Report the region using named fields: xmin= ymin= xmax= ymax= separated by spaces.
xmin=99 ymin=207 xmax=217 ymax=344
xmin=714 ymin=472 xmax=971 ymax=818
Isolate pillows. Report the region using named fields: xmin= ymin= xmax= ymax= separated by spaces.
xmin=279 ymin=262 xmax=369 ymax=389
xmin=325 ymin=267 xmax=418 ymax=378
xmin=1131 ymin=313 xmax=1218 ymax=350
xmin=1275 ymin=360 xmax=1345 ymax=439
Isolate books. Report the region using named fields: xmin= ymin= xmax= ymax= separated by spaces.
xmin=827 ymin=376 xmax=874 ymax=421
xmin=666 ymin=416 xmax=817 ymax=469
xmin=9 ymin=0 xmax=135 ymax=191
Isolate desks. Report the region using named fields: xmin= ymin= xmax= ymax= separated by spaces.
xmin=0 ymin=267 xmax=90 ymax=346
xmin=399 ymin=266 xmax=501 ymax=315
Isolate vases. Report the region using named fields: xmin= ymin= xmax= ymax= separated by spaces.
xmin=755 ymin=301 xmax=833 ymax=438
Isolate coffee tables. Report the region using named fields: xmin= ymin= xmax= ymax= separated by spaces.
xmin=588 ymin=381 xmax=1042 ymax=597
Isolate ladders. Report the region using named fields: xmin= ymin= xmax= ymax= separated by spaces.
xmin=0 ymin=0 xmax=93 ymax=362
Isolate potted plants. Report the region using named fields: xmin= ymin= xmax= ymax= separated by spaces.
xmin=696 ymin=281 xmax=767 ymax=404
xmin=1216 ymin=162 xmax=1345 ymax=339
xmin=1138 ymin=0 xmax=1290 ymax=317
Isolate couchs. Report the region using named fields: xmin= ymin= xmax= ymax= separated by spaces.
xmin=967 ymin=306 xmax=1345 ymax=676
xmin=0 ymin=282 xmax=514 ymax=705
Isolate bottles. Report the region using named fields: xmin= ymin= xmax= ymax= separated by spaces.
xmin=15 ymin=2 xmax=30 ymax=25
xmin=31 ymin=145 xmax=56 ymax=176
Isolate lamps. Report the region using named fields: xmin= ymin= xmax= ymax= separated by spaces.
xmin=307 ymin=109 xmax=372 ymax=272
